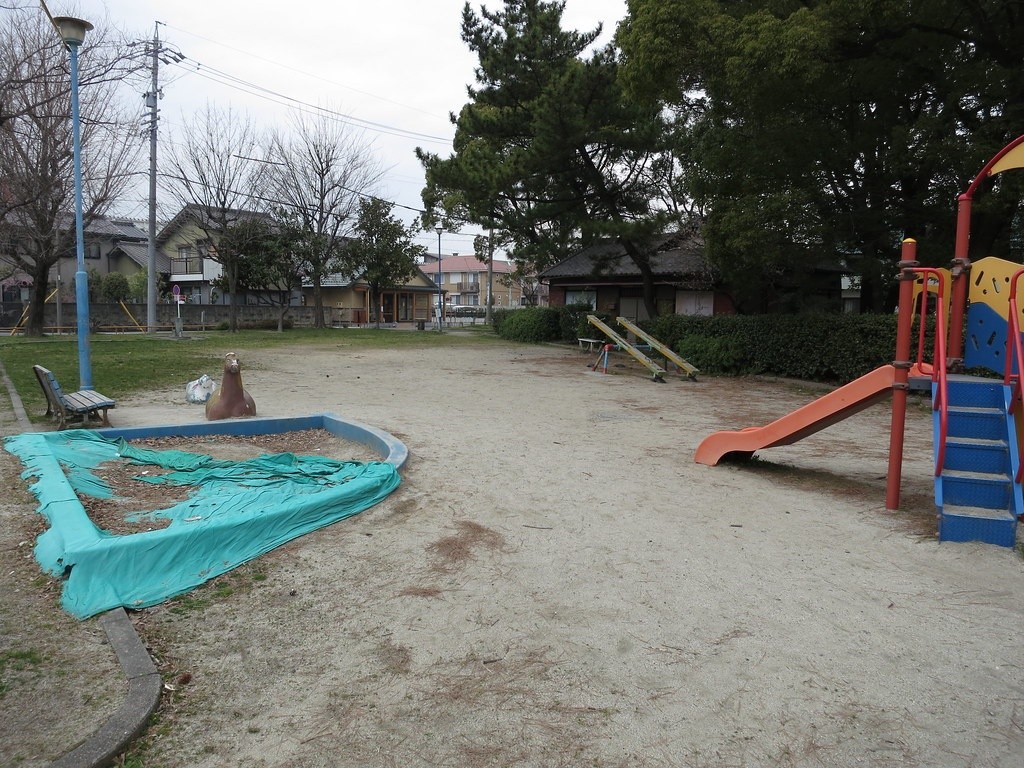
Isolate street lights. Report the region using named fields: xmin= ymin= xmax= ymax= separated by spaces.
xmin=436 ymin=227 xmax=443 ymax=333
xmin=51 ymin=16 xmax=96 ymax=393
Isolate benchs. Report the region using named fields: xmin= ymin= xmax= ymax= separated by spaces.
xmin=32 ymin=365 xmax=115 ymax=430
xmin=578 ymin=338 xmax=605 ymax=355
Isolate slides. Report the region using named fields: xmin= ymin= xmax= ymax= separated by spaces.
xmin=693 ymin=361 xmax=937 ymax=467
